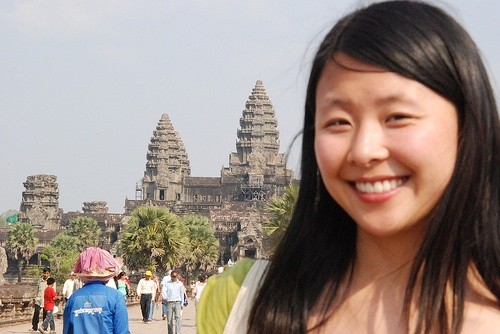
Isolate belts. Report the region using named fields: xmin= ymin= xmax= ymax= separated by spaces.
xmin=168 ymin=301 xmax=181 ymax=303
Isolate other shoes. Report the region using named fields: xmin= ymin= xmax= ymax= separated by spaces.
xmin=145 ymin=321 xmax=148 ymax=324
xmin=29 ymin=329 xmax=35 ymax=331
xmin=39 ymin=327 xmax=45 ymax=334
xmin=163 ymin=315 xmax=167 ymax=320
xmin=50 ymin=330 xmax=56 ymax=333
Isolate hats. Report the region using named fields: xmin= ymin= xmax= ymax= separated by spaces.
xmin=73 ymin=246 xmax=119 ymax=282
xmin=146 ymin=271 xmax=152 ymax=276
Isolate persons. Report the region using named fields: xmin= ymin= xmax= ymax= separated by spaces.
xmin=32 ymin=268 xmax=84 ymax=334
xmin=62 ymin=245 xmax=130 ymax=334
xmin=117 ymin=271 xmax=208 ymax=334
xmin=194 ymin=0 xmax=500 ymax=334
